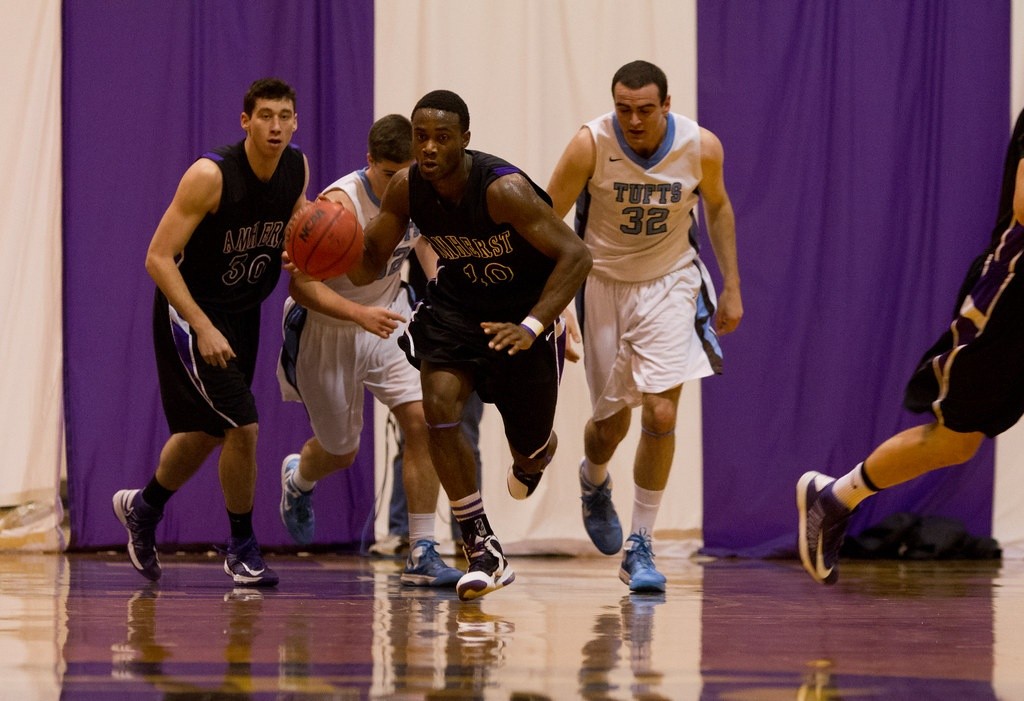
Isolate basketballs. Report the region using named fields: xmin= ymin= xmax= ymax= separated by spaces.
xmin=283 ymin=200 xmax=366 ymax=280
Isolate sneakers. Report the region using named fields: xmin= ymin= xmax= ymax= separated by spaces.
xmin=504 ymin=429 xmax=558 ymax=500
xmin=618 ymin=528 xmax=666 ymax=592
xmin=223 ymin=538 xmax=280 ymax=587
xmin=795 ymin=470 xmax=856 ymax=586
xmin=111 ymin=488 xmax=163 ymax=581
xmin=456 ymin=530 xmax=516 ymax=602
xmin=400 ymin=538 xmax=465 ymax=587
xmin=368 ymin=533 xmax=400 ymax=557
xmin=579 ymin=457 xmax=624 ymax=555
xmin=280 ymin=452 xmax=315 ymax=545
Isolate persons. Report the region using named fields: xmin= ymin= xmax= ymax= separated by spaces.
xmin=545 ymin=60 xmax=744 ymax=593
xmin=305 ymin=89 xmax=594 ymax=601
xmin=274 ymin=113 xmax=470 ymax=590
xmin=790 ymin=106 xmax=1024 ymax=585
xmin=110 ymin=79 xmax=311 ymax=589
